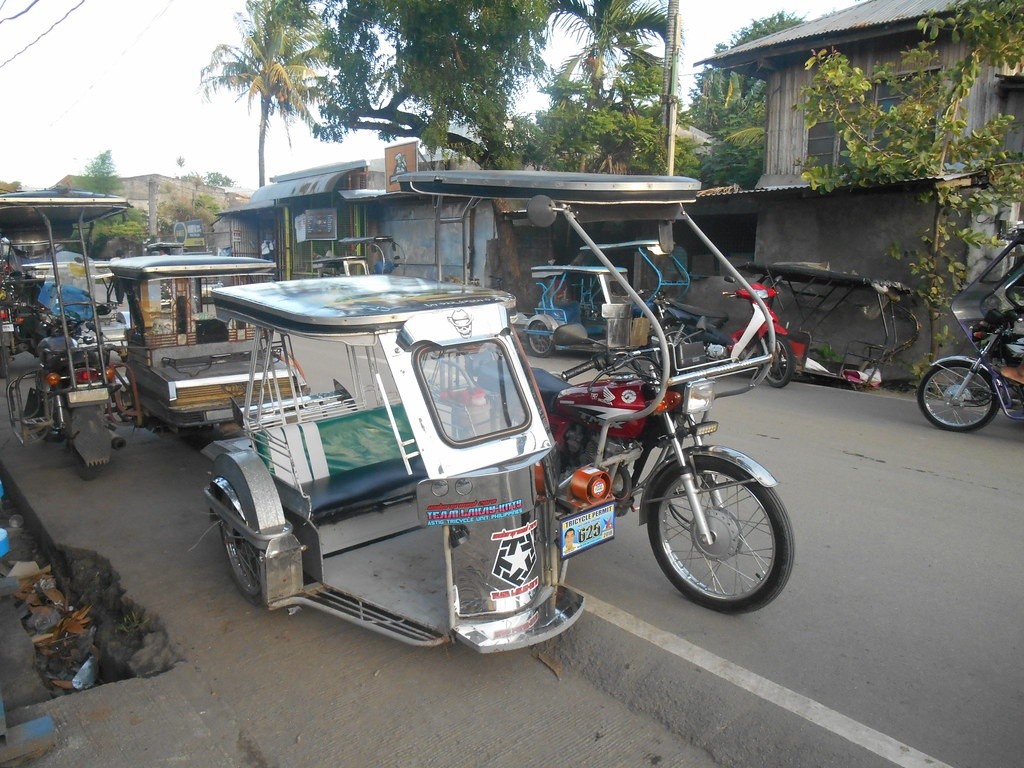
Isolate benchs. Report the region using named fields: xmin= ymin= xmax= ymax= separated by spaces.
xmin=261 ymin=398 xmax=429 ymax=556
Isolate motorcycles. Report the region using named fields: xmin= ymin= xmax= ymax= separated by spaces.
xmin=648 ymin=275 xmax=797 ymax=389
xmin=0 ymin=189 xmax=312 ymax=482
xmin=199 ymin=166 xmax=795 ymax=652
xmin=915 ymin=226 xmax=1024 ymax=434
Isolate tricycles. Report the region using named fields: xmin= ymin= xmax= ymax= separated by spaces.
xmin=523 ymin=238 xmax=694 ymax=374
